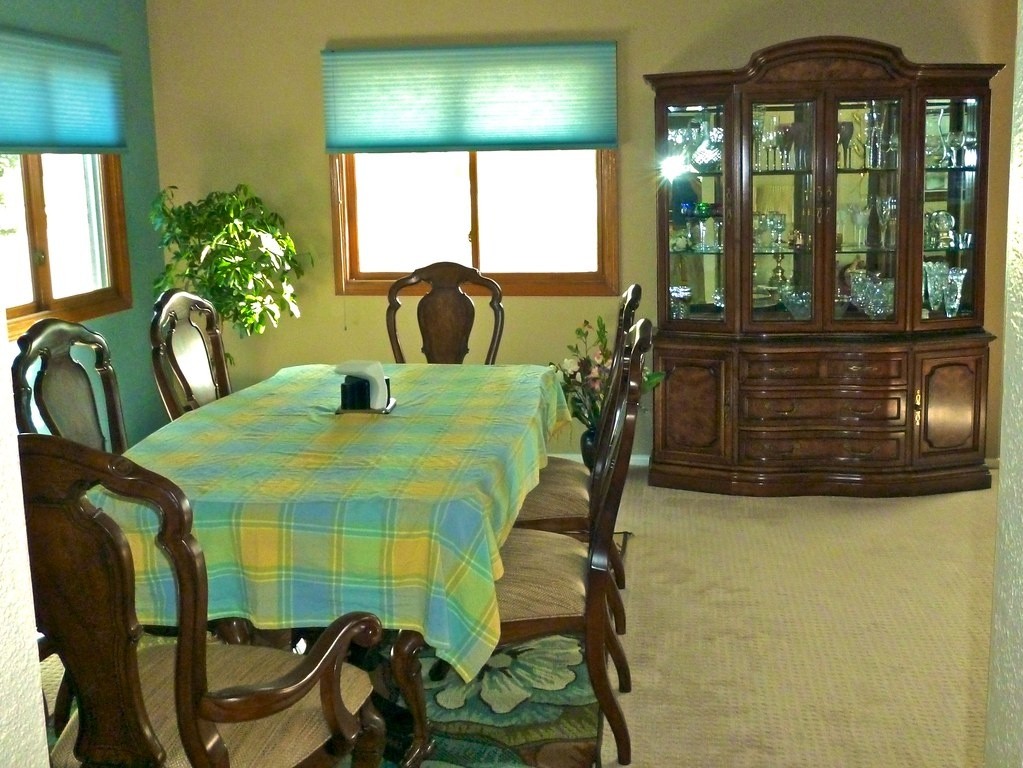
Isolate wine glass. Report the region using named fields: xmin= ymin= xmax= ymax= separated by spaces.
xmin=680 ymin=203 xmax=724 ymax=253
xmin=836 ymin=191 xmax=895 ymax=249
xmin=753 ymin=211 xmax=789 ymax=250
xmin=838 ymin=121 xmax=855 ymax=170
xmin=889 ymin=106 xmax=899 ymax=152
xmin=966 ymin=132 xmax=976 ymax=168
xmin=711 ymin=288 xmax=725 ymax=318
xmin=926 ymin=130 xmax=963 ymax=168
xmin=752 ymin=102 xmax=809 ymax=172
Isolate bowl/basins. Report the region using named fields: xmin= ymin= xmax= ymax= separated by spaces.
xmin=781 ymin=291 xmax=811 ymax=319
xmin=835 ymin=288 xmax=852 ymax=320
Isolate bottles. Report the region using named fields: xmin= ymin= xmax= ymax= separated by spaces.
xmin=865 ymin=100 xmax=886 ymax=169
xmin=691 ymin=107 xmax=721 ymax=174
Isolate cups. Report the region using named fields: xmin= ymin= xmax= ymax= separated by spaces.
xmin=850 ymin=272 xmax=895 ymax=319
xmin=924 ymin=230 xmax=938 ymax=250
xmin=923 ymin=261 xmax=967 ymax=318
xmin=958 ymin=233 xmax=972 ymax=248
xmin=670 ymin=291 xmax=692 ymax=319
xmin=669 ymin=286 xmax=691 ymax=293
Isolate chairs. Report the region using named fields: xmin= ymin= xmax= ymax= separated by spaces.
xmin=148 ymin=288 xmax=231 ymax=420
xmin=512 ymin=283 xmax=642 ymax=635
xmin=391 ymin=317 xmax=653 ymax=768
xmin=17 ymin=433 xmax=385 ymax=768
xmin=386 ymin=262 xmax=503 ymax=365
xmin=11 ymin=318 xmax=127 ymax=491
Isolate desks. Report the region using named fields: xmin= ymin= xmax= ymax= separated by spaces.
xmin=82 ymin=364 xmax=574 ymax=768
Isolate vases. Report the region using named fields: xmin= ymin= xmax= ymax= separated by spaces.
xmin=580 ymin=425 xmax=596 ymax=471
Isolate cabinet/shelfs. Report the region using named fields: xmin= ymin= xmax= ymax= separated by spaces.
xmin=642 ymin=34 xmax=1006 ymax=498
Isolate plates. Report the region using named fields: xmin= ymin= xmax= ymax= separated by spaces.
xmin=751 ymin=301 xmax=780 ymax=308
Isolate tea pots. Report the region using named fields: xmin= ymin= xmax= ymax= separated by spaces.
xmin=844 ymin=258 xmax=866 ymax=286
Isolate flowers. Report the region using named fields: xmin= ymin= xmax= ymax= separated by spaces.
xmin=548 ymin=318 xmax=672 ymax=430
